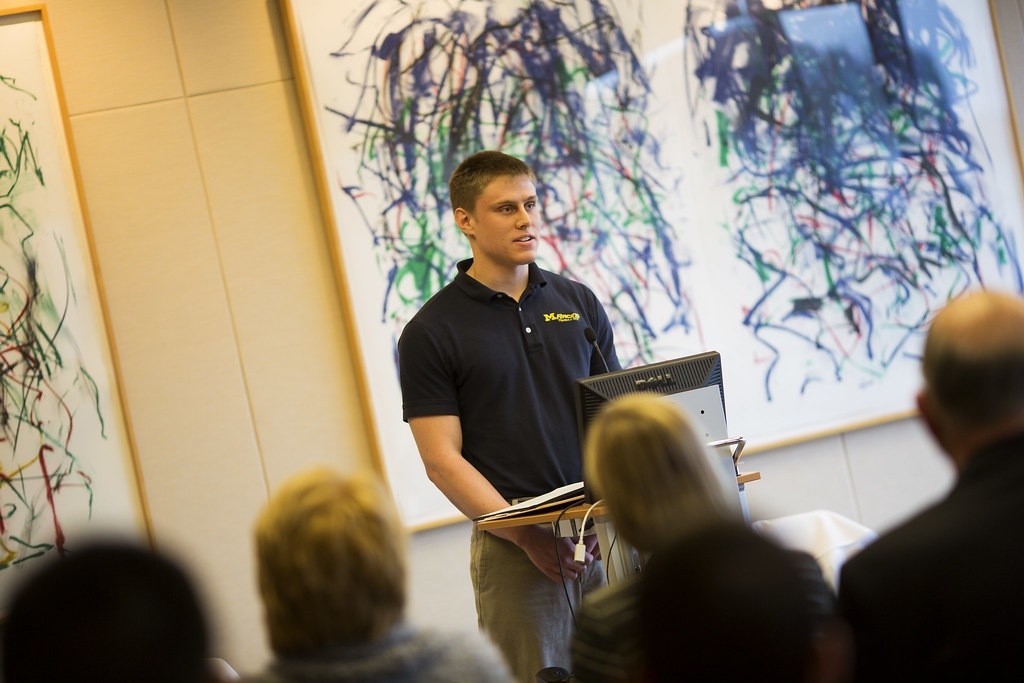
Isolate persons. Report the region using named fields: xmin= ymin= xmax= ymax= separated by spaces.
xmin=0 ymin=538 xmax=221 ymax=683
xmin=397 ymin=150 xmax=629 ymax=682
xmin=240 ymin=468 xmax=516 ymax=682
xmin=837 ymin=287 xmax=1024 ymax=683
xmin=570 ymin=390 xmax=856 ymax=683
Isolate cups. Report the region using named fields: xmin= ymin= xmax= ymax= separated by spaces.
xmin=535 ymin=667 xmax=574 ymax=683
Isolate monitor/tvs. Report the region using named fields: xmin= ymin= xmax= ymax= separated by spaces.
xmin=570 ymin=349 xmax=729 ymax=506
